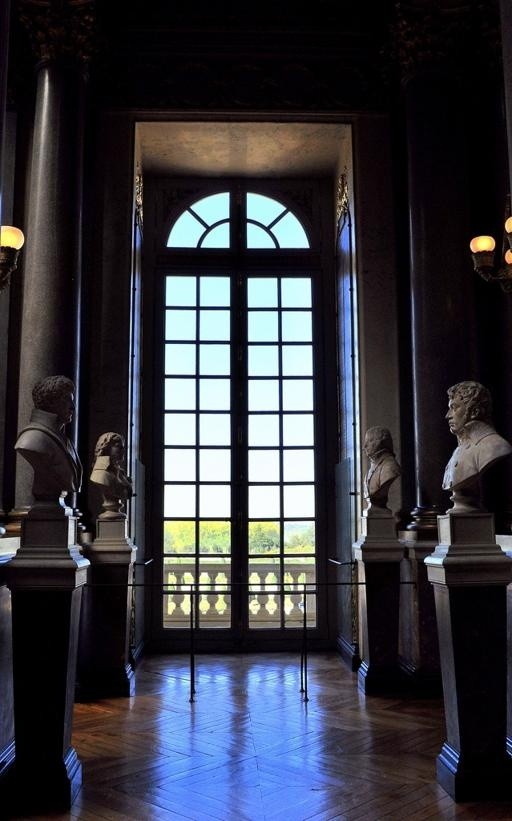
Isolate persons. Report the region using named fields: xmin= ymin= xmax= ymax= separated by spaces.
xmin=18 ymin=374 xmax=86 ymax=496
xmin=360 ymin=424 xmax=397 ymax=495
xmin=89 ymin=432 xmax=138 ymax=496
xmin=438 ymin=378 xmax=510 ymax=489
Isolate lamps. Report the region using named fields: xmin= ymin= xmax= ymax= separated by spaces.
xmin=0 ymin=224 xmax=26 ymax=291
xmin=468 ymin=192 xmax=512 ymax=289
